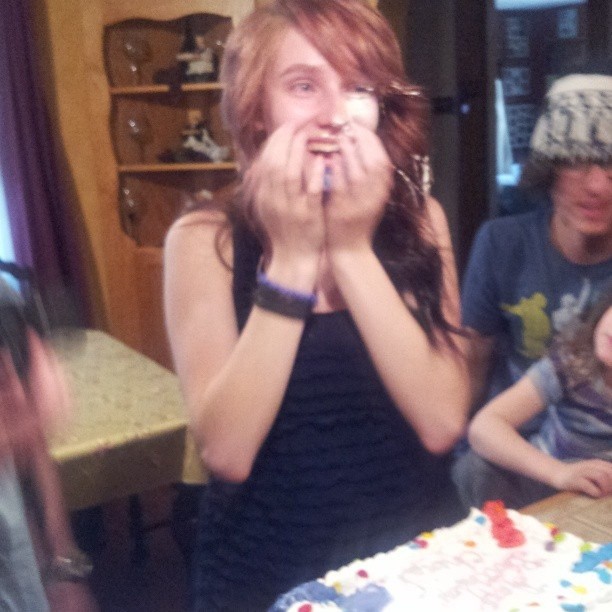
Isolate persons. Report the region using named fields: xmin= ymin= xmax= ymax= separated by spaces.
xmin=453 ymin=74 xmax=612 ymax=519
xmin=161 ymin=1 xmax=469 ymax=611
xmin=0 ymin=254 xmax=99 ymax=612
xmin=464 ymin=288 xmax=612 ymax=499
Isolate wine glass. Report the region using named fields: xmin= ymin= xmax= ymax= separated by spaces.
xmin=120 ymin=32 xmax=150 ymax=86
xmin=119 ymin=186 xmax=144 ymax=248
xmin=123 ymin=110 xmax=153 ymax=164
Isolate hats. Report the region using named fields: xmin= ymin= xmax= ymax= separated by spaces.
xmin=527 ymin=73 xmax=612 ymax=161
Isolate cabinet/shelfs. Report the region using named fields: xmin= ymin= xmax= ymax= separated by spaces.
xmin=34 ymin=0 xmax=256 ymax=367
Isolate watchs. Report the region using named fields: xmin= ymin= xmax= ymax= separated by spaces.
xmin=60 ymin=545 xmax=92 ymax=582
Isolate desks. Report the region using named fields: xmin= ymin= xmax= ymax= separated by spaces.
xmin=36 ymin=329 xmax=186 ymax=612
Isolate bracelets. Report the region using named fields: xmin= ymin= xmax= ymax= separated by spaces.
xmin=251 ymin=273 xmax=315 ymax=322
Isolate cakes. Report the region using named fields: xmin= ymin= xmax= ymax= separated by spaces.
xmin=266 ymin=499 xmax=612 ymax=612
